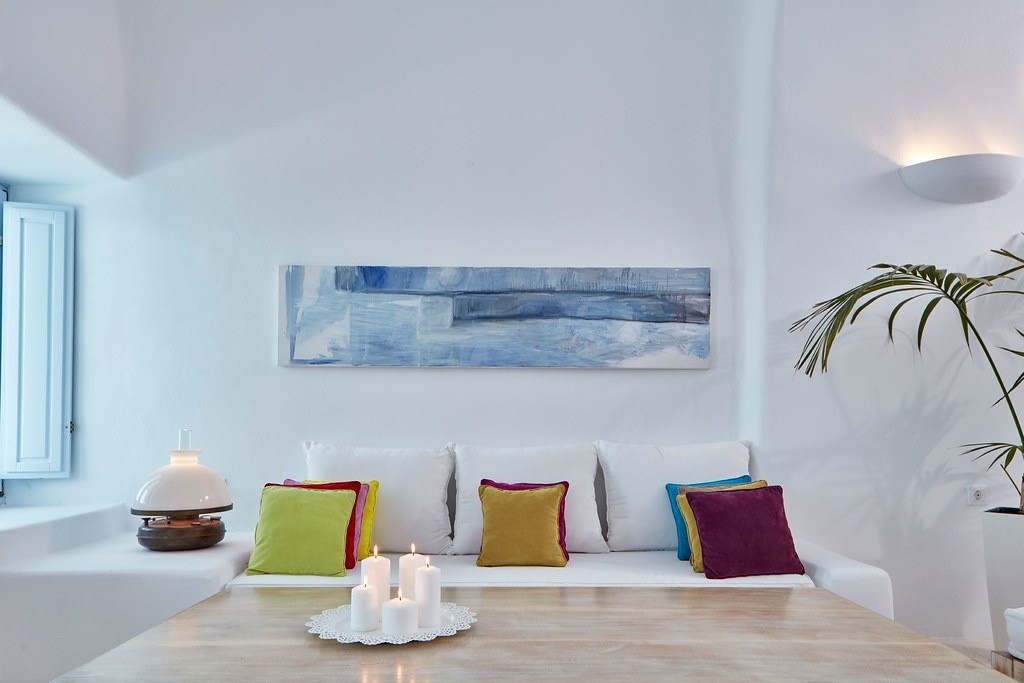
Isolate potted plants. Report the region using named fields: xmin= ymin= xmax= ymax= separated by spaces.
xmin=788 ymin=227 xmax=1023 ymax=680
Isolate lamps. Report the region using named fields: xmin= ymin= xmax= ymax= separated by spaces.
xmin=130 ymin=426 xmax=234 ymax=552
xmin=899 ymin=153 xmax=1021 ymax=204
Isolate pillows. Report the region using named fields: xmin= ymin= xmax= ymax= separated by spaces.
xmin=676 ymin=479 xmax=768 ymax=573
xmin=302 ymin=440 xmax=456 ymax=555
xmin=664 ymin=473 xmax=752 ymax=562
xmin=677 ymin=480 xmax=757 ymax=494
xmin=445 ymin=441 xmax=611 ymax=556
xmin=300 ymin=480 xmax=380 ymax=562
xmin=245 ymin=486 xmax=356 ymax=578
xmin=592 ymin=438 xmax=750 ymax=552
xmin=479 ymin=479 xmax=571 ymax=562
xmin=281 ymin=478 xmax=368 ymax=563
xmin=261 ymin=480 xmax=362 ymax=570
xmin=685 ymin=485 xmax=806 ymax=579
xmin=475 ymin=483 xmax=568 ymax=568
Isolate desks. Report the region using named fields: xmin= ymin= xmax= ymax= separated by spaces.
xmin=52 ymin=585 xmax=1022 ymax=683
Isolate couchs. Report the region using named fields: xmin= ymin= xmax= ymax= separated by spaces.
xmin=224 ymin=441 xmax=896 ymax=624
